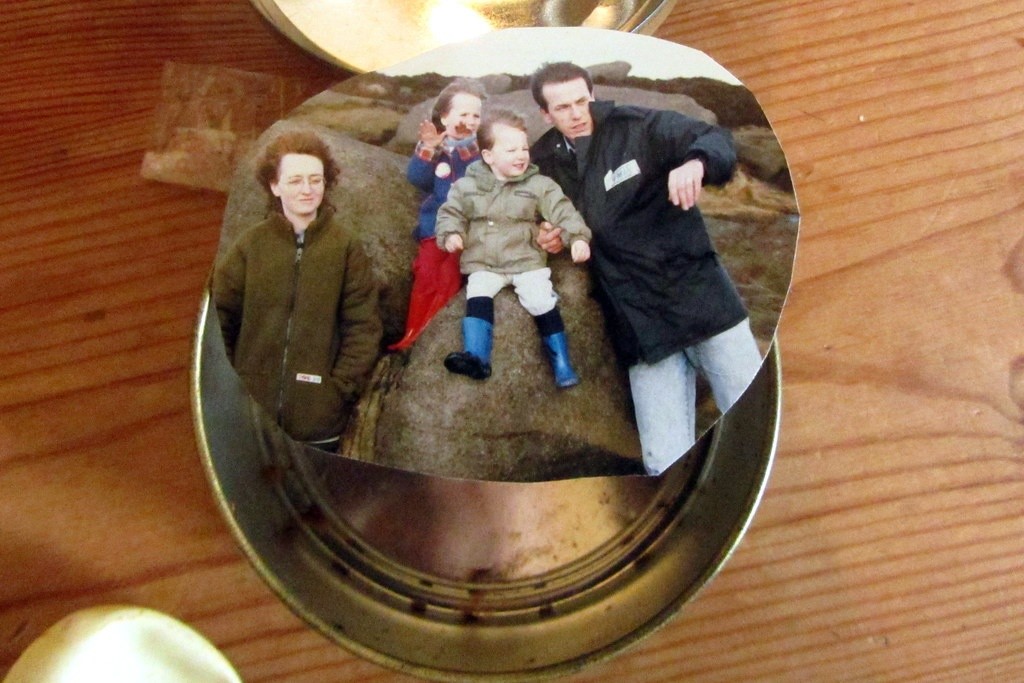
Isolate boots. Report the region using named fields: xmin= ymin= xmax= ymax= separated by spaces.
xmin=443 ymin=316 xmax=493 ymax=380
xmin=542 ymin=331 xmax=583 ymax=390
xmin=388 ymin=283 xmax=449 ymax=350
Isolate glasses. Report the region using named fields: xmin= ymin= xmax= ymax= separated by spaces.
xmin=276 ymin=174 xmax=327 ymax=186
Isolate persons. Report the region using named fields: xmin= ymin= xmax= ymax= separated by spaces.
xmin=529 ymin=64 xmax=762 ymax=475
xmin=206 ymin=130 xmax=382 ymax=455
xmin=386 ymin=78 xmax=490 ymax=351
xmin=434 ymin=110 xmax=594 ymax=389
